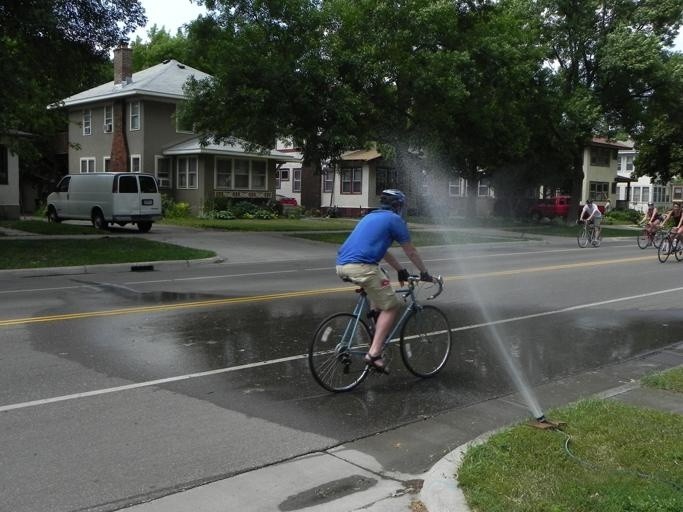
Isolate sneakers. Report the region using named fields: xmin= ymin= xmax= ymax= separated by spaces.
xmin=593 ymin=241 xmax=597 ymax=244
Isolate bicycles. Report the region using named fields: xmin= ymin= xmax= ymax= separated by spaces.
xmin=636 ymin=221 xmax=663 ymax=249
xmin=308 ymin=271 xmax=453 ymax=394
xmin=576 ymin=219 xmax=603 ymax=248
xmin=655 ymin=224 xmax=683 ymax=264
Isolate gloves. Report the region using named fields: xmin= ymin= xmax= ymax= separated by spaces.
xmin=421 ymin=271 xmax=433 ymax=283
xmin=399 ymin=269 xmax=409 ymax=281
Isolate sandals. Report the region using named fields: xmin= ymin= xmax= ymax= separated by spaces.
xmin=363 ymin=352 xmax=390 ymax=374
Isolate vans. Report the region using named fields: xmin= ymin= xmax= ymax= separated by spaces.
xmin=45 ymin=171 xmax=162 ymax=231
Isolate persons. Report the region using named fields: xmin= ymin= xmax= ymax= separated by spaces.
xmin=580 ymin=199 xmax=602 ymax=245
xmin=640 ymin=201 xmax=683 ymax=255
xmin=604 ymin=199 xmax=612 ymax=217
xmin=336 ymin=189 xmax=435 ymax=375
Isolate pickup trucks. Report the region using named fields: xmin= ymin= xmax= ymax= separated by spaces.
xmin=528 ymin=196 xmax=605 ymax=225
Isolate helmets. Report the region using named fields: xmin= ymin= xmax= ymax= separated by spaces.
xmin=587 ymin=199 xmax=593 ymax=204
xmin=381 ymin=189 xmax=406 ymax=210
xmin=648 ymin=202 xmax=654 ymax=208
